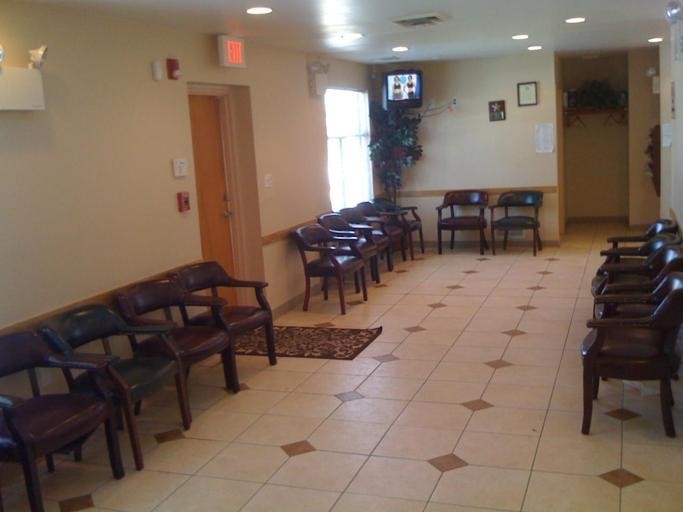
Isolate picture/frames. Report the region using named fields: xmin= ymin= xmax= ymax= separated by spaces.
xmin=517 ymin=81 xmax=537 ymax=106
xmin=488 ymin=100 xmax=505 ymax=120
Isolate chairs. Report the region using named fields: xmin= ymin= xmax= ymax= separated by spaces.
xmin=581 ymin=216 xmax=683 ymax=438
xmin=435 ymin=189 xmax=488 ymax=255
xmin=168 ymin=259 xmax=277 ymax=388
xmin=35 ymin=303 xmax=193 ymax=471
xmin=1 ymin=329 xmax=126 ymax=512
xmin=291 ymin=198 xmax=424 ymax=315
xmin=488 ymin=191 xmax=542 ymax=257
xmin=112 ymin=277 xmax=239 ymax=395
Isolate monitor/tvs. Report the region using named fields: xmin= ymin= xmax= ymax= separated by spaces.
xmin=382 ymin=69 xmax=423 ymax=110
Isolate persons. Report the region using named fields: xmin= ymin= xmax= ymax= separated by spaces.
xmin=404 ymin=75 xmax=416 ymax=99
xmin=393 ymin=76 xmax=403 ymax=100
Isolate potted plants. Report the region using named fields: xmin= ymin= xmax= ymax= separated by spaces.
xmin=368 ymin=101 xmax=423 ymax=251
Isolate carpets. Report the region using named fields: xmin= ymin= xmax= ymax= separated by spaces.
xmin=217 ymin=323 xmax=383 ymax=360
